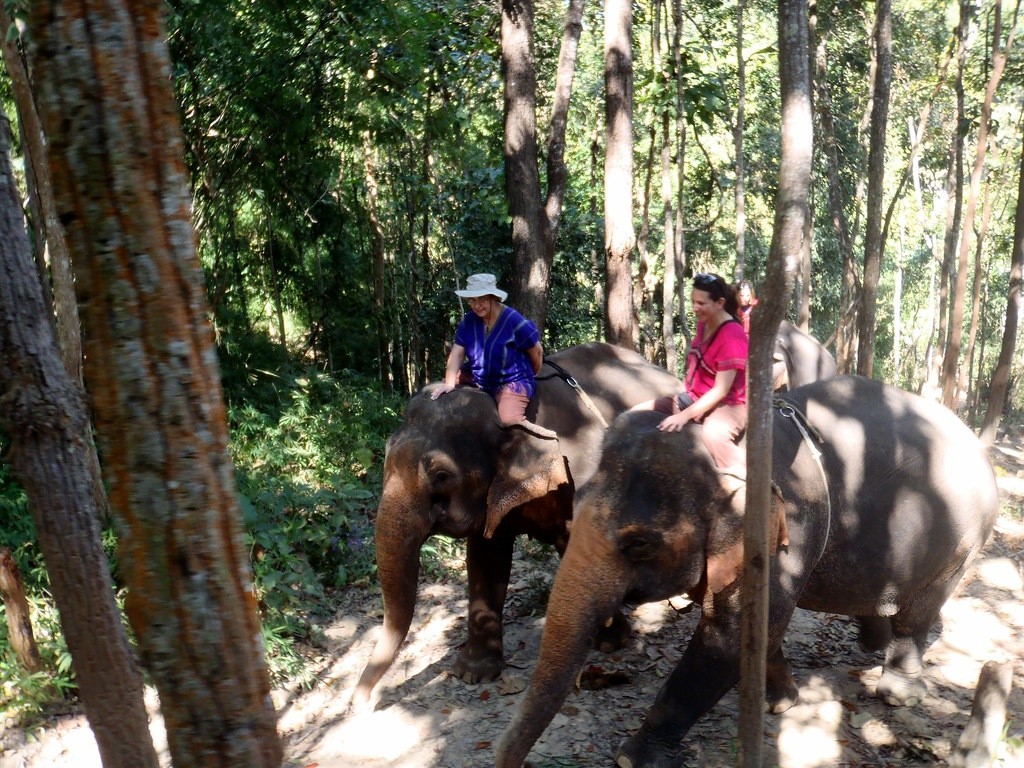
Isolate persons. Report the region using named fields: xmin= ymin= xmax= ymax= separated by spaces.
xmin=430 ymin=272 xmax=557 ymax=440
xmin=630 ymin=272 xmax=746 ymax=471
xmin=735 ymin=279 xmax=756 ymax=338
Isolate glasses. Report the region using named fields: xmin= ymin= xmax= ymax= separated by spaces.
xmin=694 ymin=274 xmax=725 ymax=298
xmin=466 ymin=295 xmax=495 ymax=305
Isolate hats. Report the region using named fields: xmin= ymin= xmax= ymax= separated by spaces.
xmin=454 ymin=273 xmax=508 ymax=302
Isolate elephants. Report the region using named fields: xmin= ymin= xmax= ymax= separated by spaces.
xmin=351 ymin=317 xmax=1000 ymax=768
